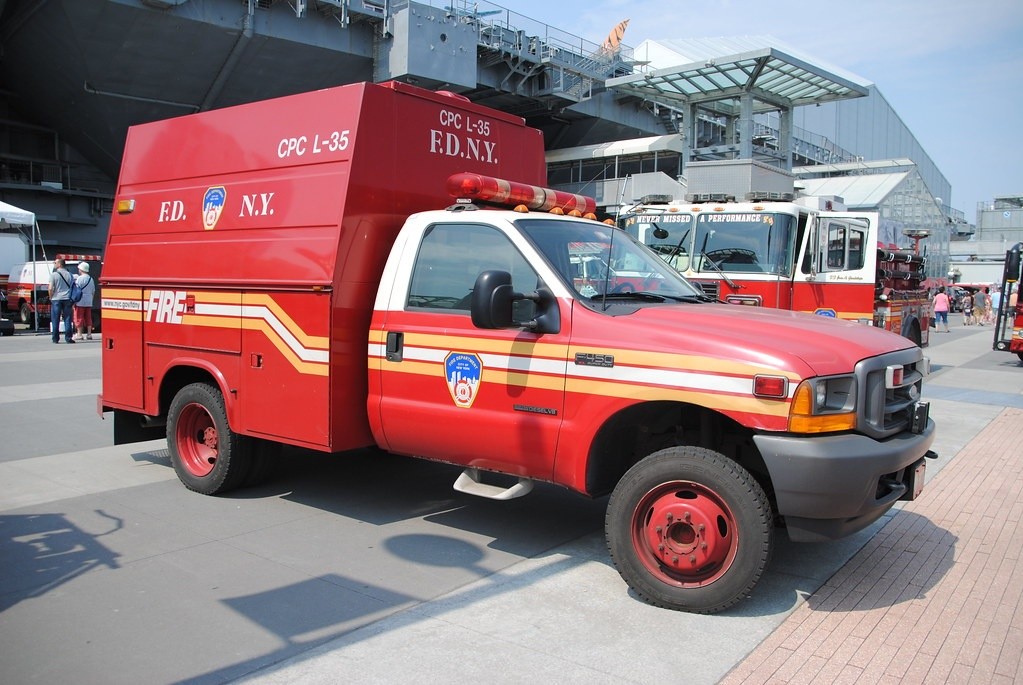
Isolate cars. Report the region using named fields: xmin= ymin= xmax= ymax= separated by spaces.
xmin=948 ymin=290 xmax=969 ymax=313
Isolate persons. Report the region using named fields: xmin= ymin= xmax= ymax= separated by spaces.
xmin=74 ymin=262 xmax=95 ymax=339
xmin=1008 ymin=289 xmax=1018 ymax=328
xmin=932 ymin=286 xmax=965 ymax=332
xmin=48 ymin=259 xmax=75 ymax=343
xmin=962 ymin=287 xmax=1007 ymax=326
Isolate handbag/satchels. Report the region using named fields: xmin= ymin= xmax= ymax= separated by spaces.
xmin=71 ymin=282 xmax=82 ymax=302
xmin=69 ymin=298 xmax=74 ymax=308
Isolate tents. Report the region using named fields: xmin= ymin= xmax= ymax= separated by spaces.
xmin=0 ymin=201 xmax=37 ymax=331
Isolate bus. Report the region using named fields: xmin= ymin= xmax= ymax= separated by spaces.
xmin=948 ymin=284 xmax=994 ymax=310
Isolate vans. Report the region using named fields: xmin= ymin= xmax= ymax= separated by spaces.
xmin=7 ymin=253 xmax=102 ymax=332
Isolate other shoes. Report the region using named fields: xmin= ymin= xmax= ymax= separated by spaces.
xmin=86 ymin=335 xmax=92 ymax=340
xmin=53 ymin=340 xmax=58 ymax=344
xmin=72 ymin=333 xmax=83 ymax=340
xmin=945 ymin=330 xmax=949 ymax=333
xmin=66 ymin=339 xmax=75 ymax=343
xmin=935 ymin=330 xmax=939 ymax=333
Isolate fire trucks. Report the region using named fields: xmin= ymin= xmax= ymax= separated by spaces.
xmin=570 ymin=200 xmax=935 ymax=355
xmin=992 ymin=241 xmax=1023 ymax=363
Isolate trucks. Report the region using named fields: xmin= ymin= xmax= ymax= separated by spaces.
xmin=96 ymin=79 xmax=937 ymax=616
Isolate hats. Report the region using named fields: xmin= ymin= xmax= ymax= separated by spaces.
xmin=78 ymin=262 xmax=90 ymax=272
